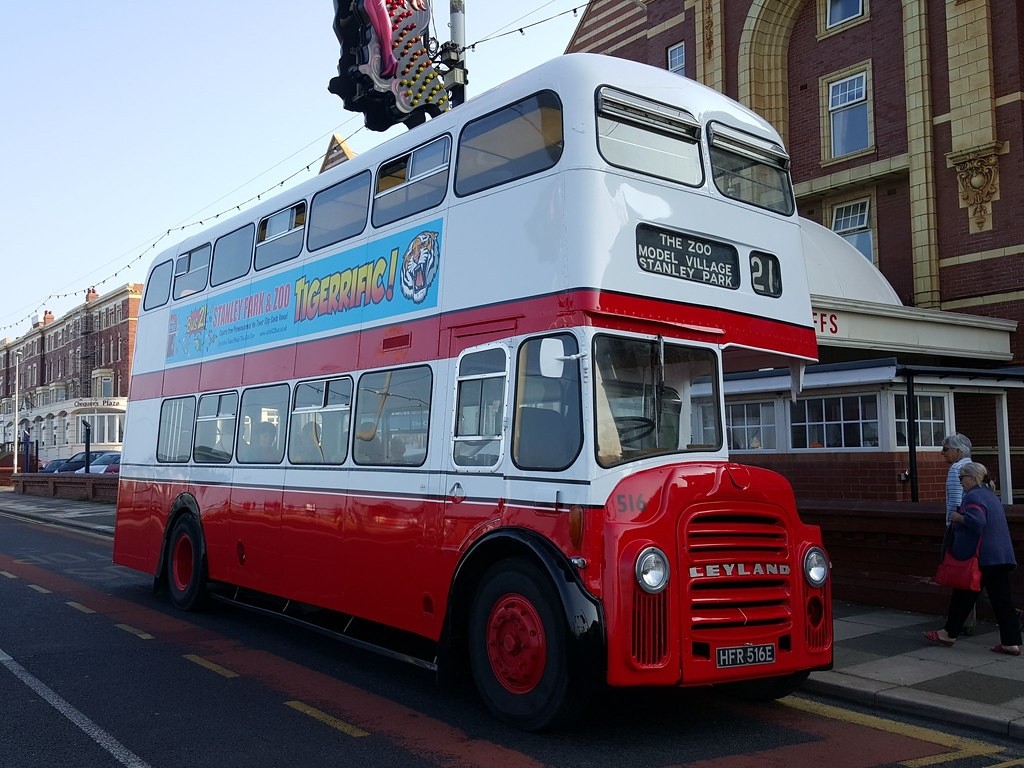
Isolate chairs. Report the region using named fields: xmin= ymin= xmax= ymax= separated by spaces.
xmin=454 ymin=454 xmax=476 ymax=466
xmin=518 ymin=407 xmax=571 ymax=470
xmin=477 ymin=454 xmax=500 ymax=466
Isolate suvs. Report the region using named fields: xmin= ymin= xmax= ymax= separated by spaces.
xmin=0 ymin=452 xmax=43 ymax=486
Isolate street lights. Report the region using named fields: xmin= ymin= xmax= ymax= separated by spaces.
xmin=12 ymin=352 xmax=23 ymax=471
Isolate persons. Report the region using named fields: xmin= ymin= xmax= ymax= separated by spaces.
xmin=933 ymin=432 xmax=976 ymax=636
xmin=922 ymin=464 xmax=1024 ymax=657
xmin=296 ymin=422 xmax=323 ymax=462
xmin=243 ymin=420 xmax=277 ymax=462
xmin=212 ymin=419 xmax=248 ymax=460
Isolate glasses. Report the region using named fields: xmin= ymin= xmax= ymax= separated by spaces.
xmin=942 ymin=447 xmax=956 ymax=452
xmin=958 ymin=474 xmax=973 ymax=481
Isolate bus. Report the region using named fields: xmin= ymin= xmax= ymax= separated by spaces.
xmin=112 ymin=53 xmax=834 ymax=731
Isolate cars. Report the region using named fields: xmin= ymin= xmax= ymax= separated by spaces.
xmin=75 ymin=451 xmax=120 ymax=473
xmin=55 ymin=451 xmax=115 ymax=472
xmin=38 ymin=459 xmax=70 ymax=472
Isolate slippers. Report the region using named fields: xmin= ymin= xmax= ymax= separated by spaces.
xmin=990 ymin=644 xmax=1021 ymax=656
xmin=922 ymin=630 xmax=954 ymax=647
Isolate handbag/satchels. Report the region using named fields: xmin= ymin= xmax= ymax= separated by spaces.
xmin=933 ymin=504 xmax=983 ymax=593
